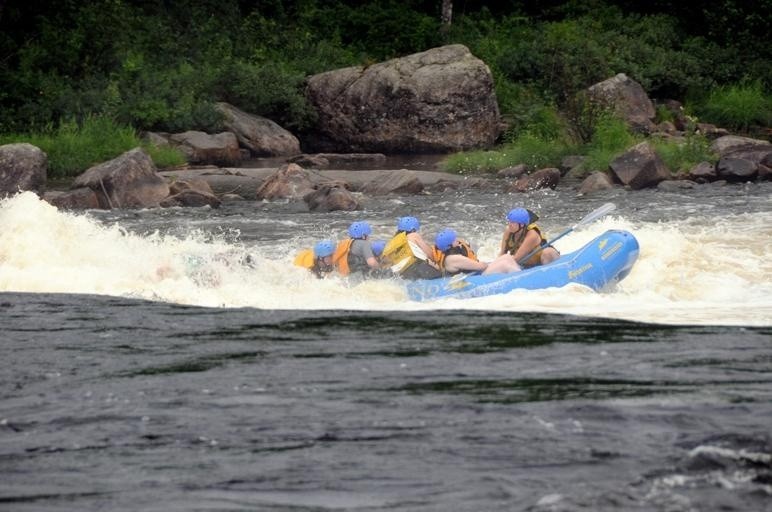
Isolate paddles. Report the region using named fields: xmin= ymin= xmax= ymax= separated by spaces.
xmin=519 ymin=202 xmax=616 ymax=262
xmin=391 ymin=240 xmax=429 ymax=273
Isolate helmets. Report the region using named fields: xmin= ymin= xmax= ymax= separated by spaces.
xmin=313 ymin=240 xmax=335 ymax=257
xmin=506 ymin=208 xmax=529 ymax=225
xmin=348 ymin=222 xmax=372 ymax=239
xmin=398 ymin=216 xmax=419 ymax=232
xmin=434 ymin=228 xmax=460 ymax=252
xmin=371 ymin=240 xmax=385 ymax=256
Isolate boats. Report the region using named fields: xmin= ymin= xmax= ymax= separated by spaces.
xmin=346 ymin=227 xmax=642 ymax=306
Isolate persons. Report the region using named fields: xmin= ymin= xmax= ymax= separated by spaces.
xmin=501 ymin=208 xmax=559 ymax=266
xmin=304 ymin=215 xmax=521 ymax=281
